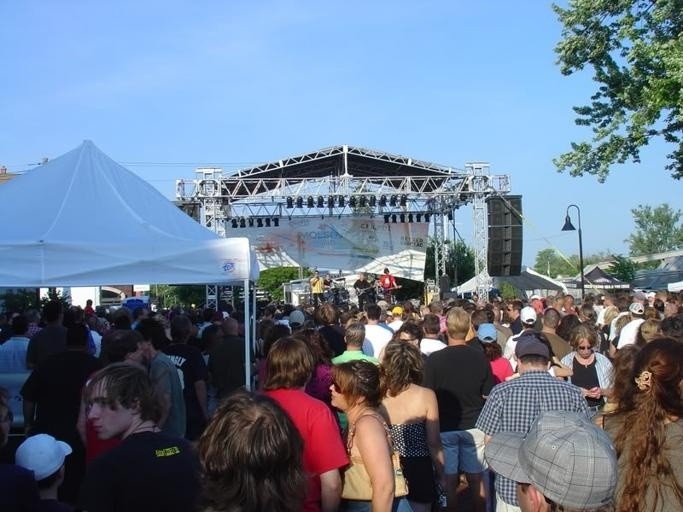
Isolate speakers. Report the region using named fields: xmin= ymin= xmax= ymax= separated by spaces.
xmin=178 ymin=204 xmax=200 ymax=224
xmin=442 ymin=291 xmax=457 ymax=301
xmin=485 ymin=195 xmax=523 ymax=277
xmin=440 ymin=277 xmax=450 ymax=294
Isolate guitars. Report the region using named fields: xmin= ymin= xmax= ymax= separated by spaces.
xmin=379 ymin=285 xmax=401 ymax=294
xmin=356 ymin=285 xmax=372 ymax=297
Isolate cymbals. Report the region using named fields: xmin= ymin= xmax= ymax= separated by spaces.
xmin=335 ymin=277 xmax=345 ymax=281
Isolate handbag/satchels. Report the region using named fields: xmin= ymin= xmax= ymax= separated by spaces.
xmin=342 ymin=412 xmax=409 ymax=501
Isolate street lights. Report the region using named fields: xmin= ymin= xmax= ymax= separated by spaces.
xmin=230 ymin=194 xmax=451 ymax=229
xmin=561 ymin=204 xmax=584 ymax=301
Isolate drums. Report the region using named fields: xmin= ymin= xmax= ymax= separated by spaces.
xmin=329 ymin=287 xmax=348 ymax=303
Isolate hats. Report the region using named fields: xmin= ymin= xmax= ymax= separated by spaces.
xmin=520 ymin=306 xmax=538 ymax=324
xmin=482 ymin=409 xmax=620 ymax=509
xmin=477 ymin=323 xmax=498 ymax=343
xmin=629 ymin=302 xmax=645 ymax=315
xmin=289 ymin=310 xmax=305 ymax=328
xmin=515 ymin=336 xmax=551 ymax=361
xmin=14 ymin=434 xmax=73 ymax=482
xmin=512 ymin=330 xmax=544 ymax=341
xmin=392 ymin=306 xmax=403 ymax=314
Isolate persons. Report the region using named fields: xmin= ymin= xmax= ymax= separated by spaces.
xmin=0 ymin=290 xmax=683 ymax=512
xmin=311 ymin=269 xmax=398 ymax=308
